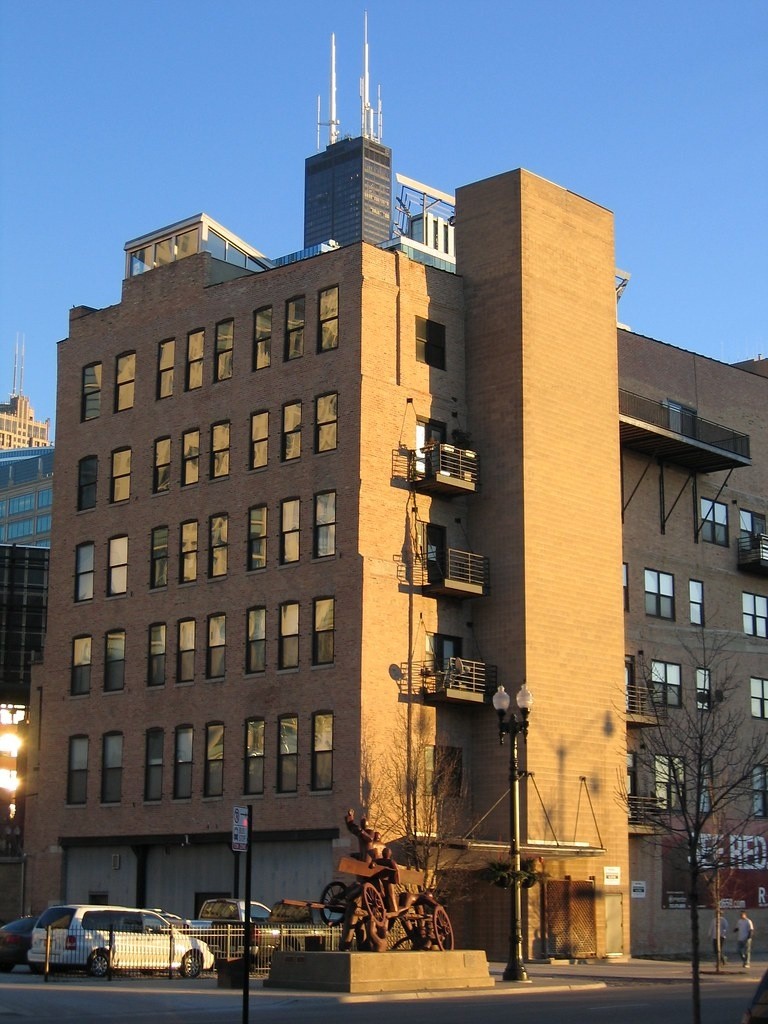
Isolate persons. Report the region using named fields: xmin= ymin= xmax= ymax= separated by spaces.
xmin=733 ymin=911 xmax=754 ymax=969
xmin=345 ymin=808 xmax=396 ymax=869
xmin=708 ymin=910 xmax=729 ymax=968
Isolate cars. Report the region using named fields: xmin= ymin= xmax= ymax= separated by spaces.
xmin=0 ymin=907 xmax=193 ymax=975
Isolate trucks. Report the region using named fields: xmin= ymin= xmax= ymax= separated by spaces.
xmin=180 ymin=899 xmax=281 ymax=961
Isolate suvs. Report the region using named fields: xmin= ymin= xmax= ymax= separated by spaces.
xmin=27 ymin=902 xmax=215 ymax=980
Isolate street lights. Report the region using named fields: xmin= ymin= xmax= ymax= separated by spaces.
xmin=493 ymin=681 xmax=534 ymax=981
